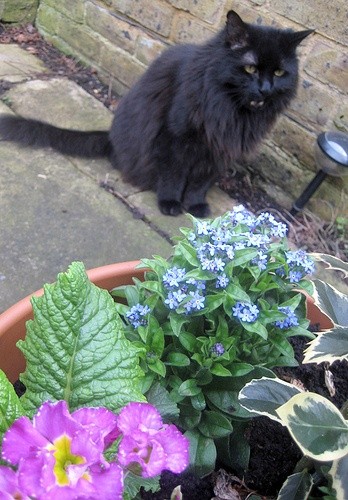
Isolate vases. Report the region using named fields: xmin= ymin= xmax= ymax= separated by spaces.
xmin=0 ymin=256 xmax=169 ymax=382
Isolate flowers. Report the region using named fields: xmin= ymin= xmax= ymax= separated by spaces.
xmin=1 ymin=202 xmax=314 ymax=498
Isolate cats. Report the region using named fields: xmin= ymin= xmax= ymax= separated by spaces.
xmin=0 ymin=9 xmax=315 ymax=220
